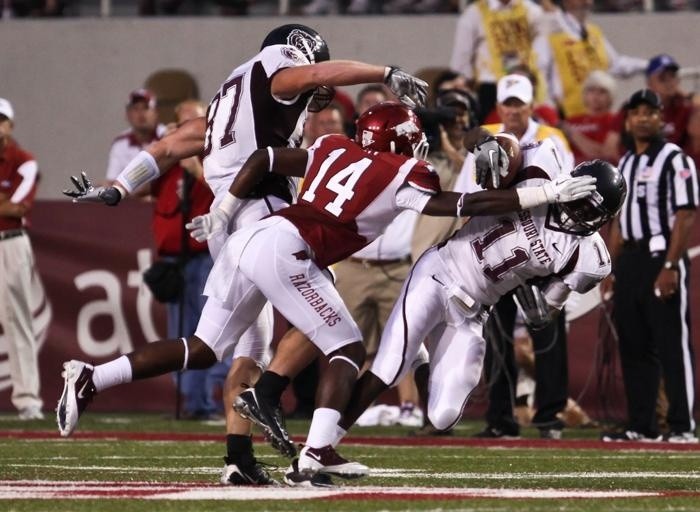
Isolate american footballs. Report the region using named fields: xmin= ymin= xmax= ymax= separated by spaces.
xmin=484 ymin=132 xmax=521 ymax=190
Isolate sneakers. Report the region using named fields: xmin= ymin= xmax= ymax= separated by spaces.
xmin=19 ymin=408 xmax=48 ymax=422
xmin=218 ymin=464 xmax=282 ymax=484
xmin=665 ymin=432 xmax=700 ymax=444
xmin=56 ymin=358 xmax=98 ymax=437
xmin=284 ymin=459 xmax=335 ymax=490
xmin=393 ymin=403 xmax=424 ymax=426
xmin=300 ymin=445 xmax=371 ymax=473
xmin=473 ymin=424 xmax=521 ymax=439
xmin=603 ymin=428 xmax=665 ymax=443
xmin=235 ymin=387 xmax=295 ymax=458
xmin=537 ymin=428 xmax=563 ymax=439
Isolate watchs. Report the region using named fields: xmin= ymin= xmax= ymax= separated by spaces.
xmin=662 ymin=260 xmax=677 ymax=269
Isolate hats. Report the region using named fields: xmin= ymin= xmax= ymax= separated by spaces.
xmin=643 ymin=54 xmax=681 ymax=79
xmin=626 ymin=90 xmax=660 ymax=110
xmin=495 ymin=76 xmax=535 ymax=105
xmin=431 ymin=92 xmax=468 ymax=109
xmin=127 ymin=90 xmax=158 ymax=106
xmin=1 ymin=99 xmax=14 ymax=123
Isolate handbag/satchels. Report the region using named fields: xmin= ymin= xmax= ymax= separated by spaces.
xmin=141 ymin=257 xmax=189 ymax=305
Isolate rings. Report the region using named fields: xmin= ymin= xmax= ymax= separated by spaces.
xmin=669 ymin=288 xmax=675 ymax=293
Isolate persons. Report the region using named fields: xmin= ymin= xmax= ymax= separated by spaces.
xmin=354 ymin=82 xmax=390 ymax=119
xmin=600 ymin=90 xmax=700 ymax=444
xmin=303 ymin=105 xmax=346 ymax=145
xmin=0 ymin=96 xmax=44 ymax=422
xmin=450 ymin=1 xmax=569 ymax=122
xmin=413 ymin=87 xmax=468 ymax=267
xmin=559 ymin=69 xmax=622 ymax=164
xmin=320 ymin=198 xmax=427 ymax=427
xmin=93 ymin=87 xmax=173 ymax=199
xmin=63 ymin=24 xmax=429 ymax=485
xmin=455 ymin=73 xmax=576 ymax=442
xmin=281 ymin=134 xmax=627 ymax=489
xmin=550 ymin=0 xmax=648 ymax=115
xmin=641 ymin=57 xmax=700 ymax=164
xmin=57 ymin=101 xmax=598 ymax=478
xmin=481 ymin=68 xmax=559 ymax=126
xmin=150 ymin=98 xmax=233 ymax=422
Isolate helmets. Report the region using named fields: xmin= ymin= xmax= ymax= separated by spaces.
xmin=352 ymin=101 xmax=429 ymax=160
xmin=259 ymin=25 xmax=335 ymax=111
xmin=552 ymin=162 xmax=627 ymax=239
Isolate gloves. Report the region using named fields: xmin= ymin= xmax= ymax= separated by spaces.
xmin=545 ymin=175 xmax=595 ymax=201
xmin=511 ymin=284 xmax=554 ymax=331
xmin=185 ymin=210 xmax=232 ymax=244
xmin=473 ymin=138 xmax=509 ymax=189
xmin=382 ymin=69 xmax=431 ymax=108
xmin=64 ymin=170 xmax=121 ymax=208
